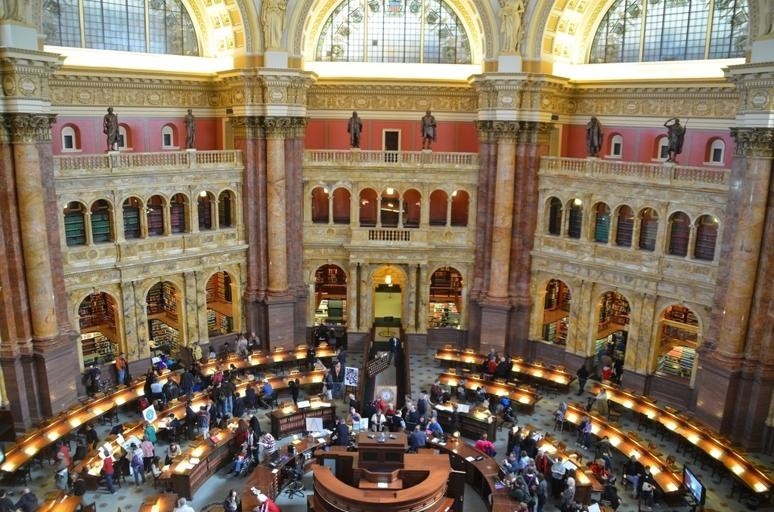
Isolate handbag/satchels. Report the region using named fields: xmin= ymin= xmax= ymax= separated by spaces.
xmin=489 ymin=447 xmax=496 ymax=457
xmin=131 ymin=455 xmax=144 ymax=468
xmin=527 ymin=500 xmax=536 ymax=508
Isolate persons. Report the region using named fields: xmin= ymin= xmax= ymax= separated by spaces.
xmin=53 ymin=424 xmax=154 ymax=496
xmin=503 ymin=429 xmax=549 ymax=512
xmin=551 ymin=400 xmax=620 ymax=512
xmin=183 ymin=109 xmax=196 ymax=149
xmin=576 ymin=345 xmax=624 ymax=416
xmin=430 ymin=379 xmax=514 ymax=456
xmin=287 ymin=457 xmax=303 ymax=486
xmin=0 ymin=488 xmax=37 ymax=511
xmin=664 ymin=116 xmax=689 ymax=162
xmin=176 ymin=497 xmax=193 ymax=512
xmin=585 ymin=116 xmax=603 ymax=156
xmin=86 ymin=352 xmax=129 ymax=396
xmin=330 ymin=392 xmax=443 ymax=451
xmin=289 ymin=323 xmax=347 ymax=405
xmin=102 ymin=107 xmax=120 ymax=152
xmin=144 ymin=363 xmax=192 ymax=464
xmin=480 ymin=347 xmax=513 ymax=383
xmin=625 ymin=457 xmax=655 ymax=511
xmin=224 ymin=489 xmax=279 ymax=512
xmin=192 ymin=331 xmax=275 ymax=476
xmin=346 ymin=111 xmax=363 ymax=148
xmin=420 ymin=109 xmax=437 ymax=150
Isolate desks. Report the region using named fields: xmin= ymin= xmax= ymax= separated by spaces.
xmin=422 ymin=343 xmax=774 ymax=512
xmin=1 ymin=345 xmax=339 ymax=512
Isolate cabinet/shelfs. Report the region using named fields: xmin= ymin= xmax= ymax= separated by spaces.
xmin=661 ymin=301 xmax=698 ymax=342
xmin=204 ymin=268 xmax=232 ymax=302
xmin=543 ymin=317 xmax=568 ymax=346
xmin=81 ymin=334 xmax=113 ymax=367
xmin=600 ymin=289 xmax=629 ymax=331
xmin=543 ymin=276 xmax=570 ymax=312
xmin=75 ymin=291 xmax=110 ymax=332
xmin=593 ymin=330 xmax=625 ymax=356
xmin=206 ymin=302 xmax=233 ymax=336
xmin=143 ymin=282 xmax=164 ymax=316
xmin=161 ymin=283 xmax=179 ymax=321
xmin=150 ymin=320 xmax=180 ymax=354
